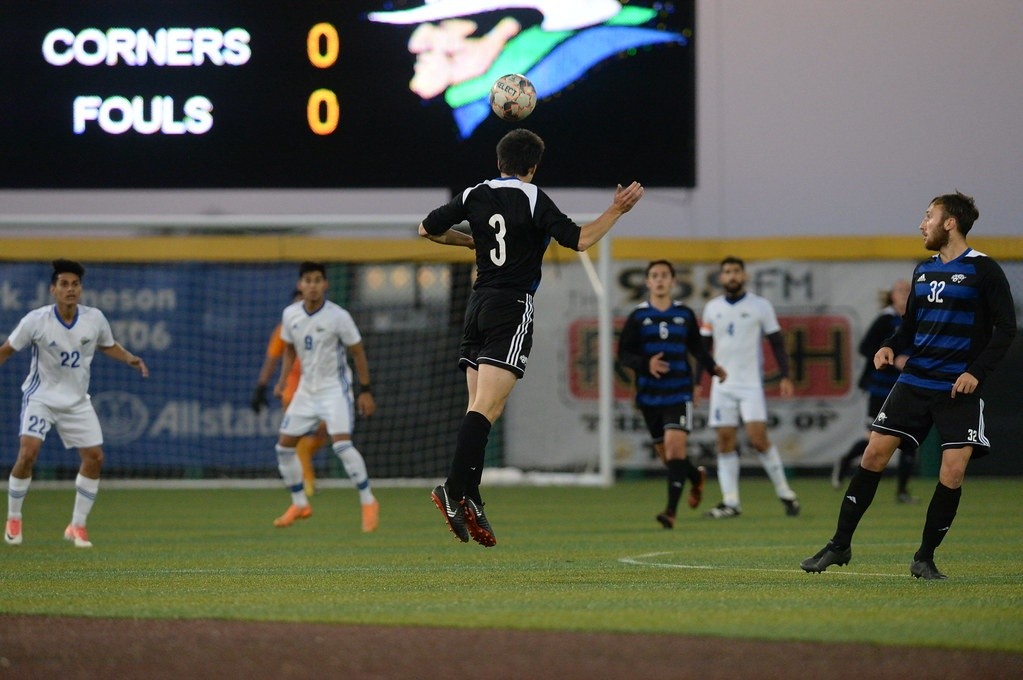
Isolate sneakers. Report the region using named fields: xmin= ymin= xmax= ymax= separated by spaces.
xmin=462 ymin=495 xmax=496 ymax=546
xmin=5 ymin=518 xmax=22 ymax=545
xmin=273 ymin=505 xmax=312 ymax=527
xmin=909 ymin=558 xmax=946 ymax=580
xmin=655 ymin=512 xmax=673 ymax=529
xmin=780 ymin=494 xmax=798 ymax=514
xmin=64 ymin=523 xmax=92 ymax=546
xmin=430 ymin=485 xmax=469 ymax=543
xmin=361 ymin=500 xmax=379 ymax=532
xmin=800 ymin=543 xmax=851 ymax=574
xmin=708 ymin=505 xmax=740 ymax=517
xmin=688 ymin=467 xmax=705 ymax=507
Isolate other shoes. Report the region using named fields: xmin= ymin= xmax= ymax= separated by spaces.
xmin=832 ymin=459 xmax=845 ymax=488
xmin=895 ymin=495 xmax=923 ymax=504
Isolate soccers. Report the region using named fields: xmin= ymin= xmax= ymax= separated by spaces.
xmin=490 ymin=74 xmax=537 ymax=122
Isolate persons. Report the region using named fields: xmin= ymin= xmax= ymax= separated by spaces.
xmin=272 ymin=262 xmax=379 ymax=532
xmin=693 ymin=257 xmax=801 ymax=518
xmin=832 ymin=279 xmax=916 ymax=504
xmin=0 ymin=260 xmax=149 ymax=546
xmin=251 ymin=289 xmax=326 ymax=495
xmin=618 ymin=260 xmax=726 ymax=529
xmin=799 ymin=193 xmax=1019 ymax=579
xmin=418 ymin=129 xmax=644 ymax=548
